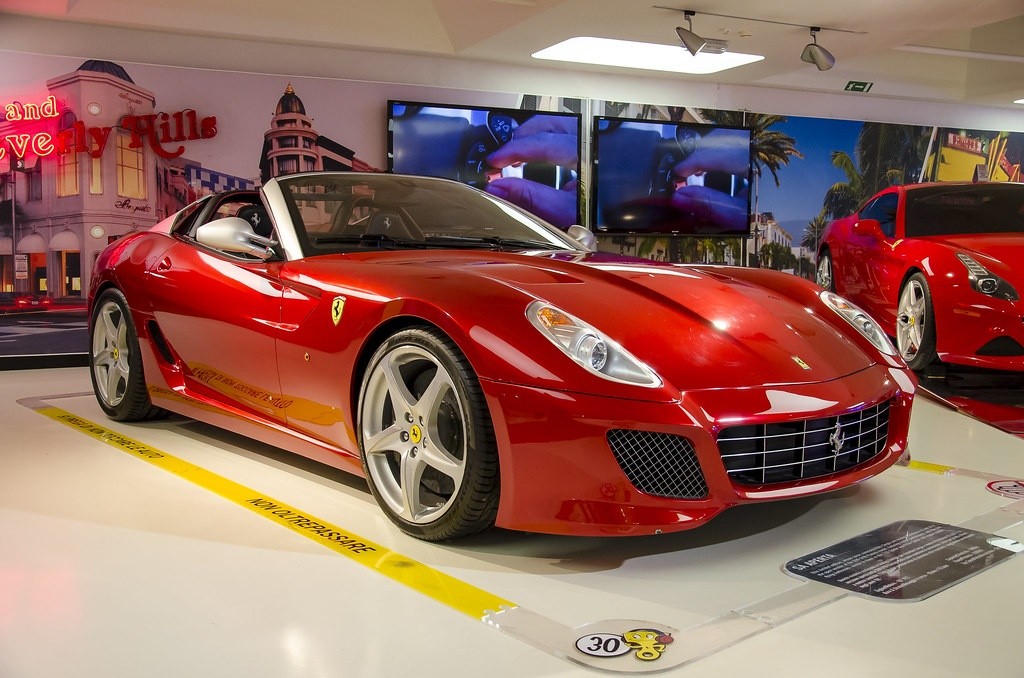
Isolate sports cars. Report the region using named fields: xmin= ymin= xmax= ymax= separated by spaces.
xmin=0 ymin=291 xmax=49 ymax=314
xmin=86 ymin=171 xmax=917 ymax=545
xmin=813 ymin=178 xmax=1024 ymax=381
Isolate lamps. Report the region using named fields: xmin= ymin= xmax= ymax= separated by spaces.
xmin=674 ymin=10 xmax=706 ymax=56
xmin=801 ymin=27 xmax=836 ymax=71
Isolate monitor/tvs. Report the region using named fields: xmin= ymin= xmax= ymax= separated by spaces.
xmin=591 ymin=115 xmax=754 ymax=239
xmin=387 ymin=100 xmax=583 ymax=231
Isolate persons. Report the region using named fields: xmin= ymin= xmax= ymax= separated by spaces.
xmin=671 ymin=129 xmax=749 ymax=232
xmin=487 ymin=115 xmax=578 ymax=229
